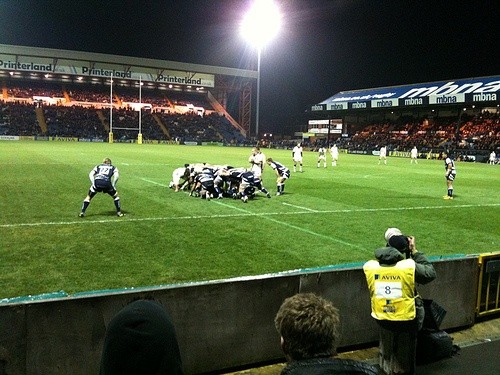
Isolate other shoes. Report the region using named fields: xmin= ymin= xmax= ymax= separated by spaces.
xmin=267 ymin=193 xmax=271 ymax=198
xmin=444 ymin=195 xmax=453 ymax=199
xmin=169 ymin=182 xmax=172 ymax=188
xmin=180 ymin=187 xmax=255 ymax=203
xmin=78 ymin=213 xmax=84 ymax=217
xmin=119 ymin=214 xmax=125 ymax=217
xmin=277 ymin=192 xmax=280 ymax=195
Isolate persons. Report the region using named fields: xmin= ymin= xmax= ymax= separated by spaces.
xmin=78 ymin=157 xmax=126 ymax=218
xmin=410 ymin=145 xmax=418 ymax=164
xmin=292 ymin=142 xmax=304 ymax=173
xmin=266 ymin=157 xmax=290 ymax=196
xmin=98 ymin=299 xmax=184 ymax=375
xmin=330 ymin=143 xmax=338 ymax=167
xmin=489 ymin=151 xmax=496 ymax=166
xmin=442 ymin=150 xmax=457 ymax=200
xmin=168 ymin=162 xmax=271 ymax=204
xmin=274 ymin=292 xmax=378 ymax=375
xmin=362 ymin=235 xmax=436 ymax=375
xmin=377 ymin=144 xmax=387 ymax=164
xmin=248 ymin=145 xmax=266 ymax=186
xmin=317 ymin=145 xmax=327 ymax=168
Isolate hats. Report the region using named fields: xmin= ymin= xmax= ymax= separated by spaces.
xmin=387 ymin=235 xmax=407 ymax=252
